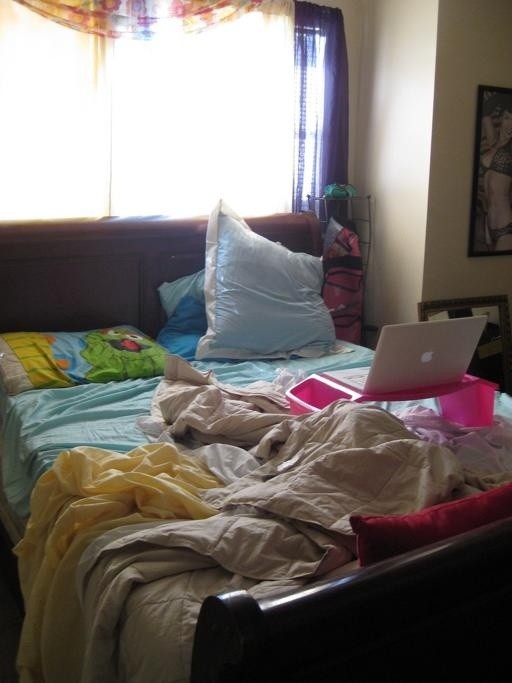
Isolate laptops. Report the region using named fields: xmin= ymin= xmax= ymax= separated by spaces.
xmin=321 ymin=315 xmax=488 ymax=396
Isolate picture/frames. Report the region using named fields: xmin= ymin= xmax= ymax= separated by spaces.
xmin=469 ymin=85 xmax=511 ymax=256
xmin=418 ymin=296 xmax=512 ymax=393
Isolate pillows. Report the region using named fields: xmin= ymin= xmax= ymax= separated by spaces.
xmin=155 ymin=199 xmax=353 ymax=363
xmin=349 ymin=482 xmax=512 ymax=566
xmin=1 ymin=323 xmax=169 ymax=397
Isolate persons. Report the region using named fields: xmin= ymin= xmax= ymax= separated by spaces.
xmin=477 ymin=87 xmax=512 ymax=252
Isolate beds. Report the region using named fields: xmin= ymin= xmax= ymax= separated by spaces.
xmin=1 ymin=211 xmax=512 ymax=683
